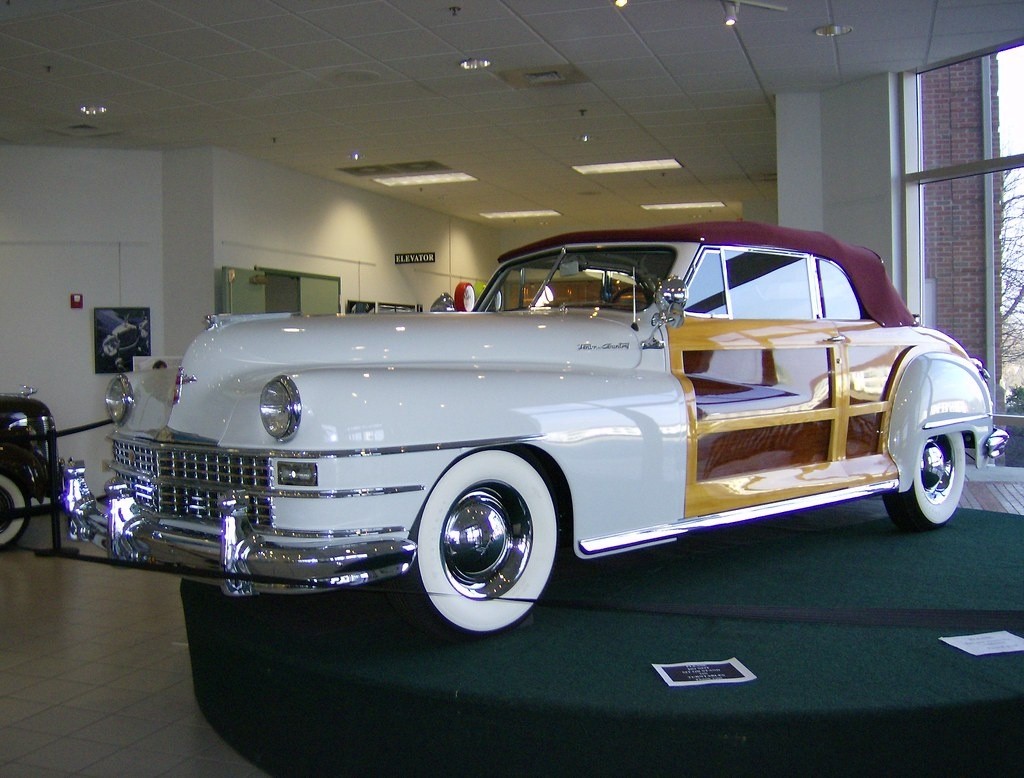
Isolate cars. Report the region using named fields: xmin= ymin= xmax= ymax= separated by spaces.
xmin=56 ymin=220 xmax=1011 ymax=640
xmin=0 ymin=383 xmax=61 ymax=553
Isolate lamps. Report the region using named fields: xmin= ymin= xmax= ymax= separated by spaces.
xmin=723 ymin=3 xmax=739 ymax=25
